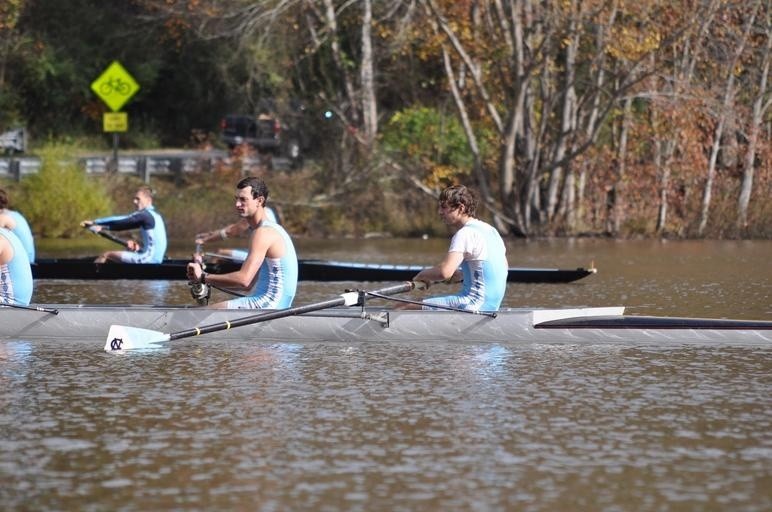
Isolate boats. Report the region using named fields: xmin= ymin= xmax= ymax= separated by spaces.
xmin=28 ymin=253 xmax=606 ymax=284
xmin=0 ymin=300 xmax=772 ymax=355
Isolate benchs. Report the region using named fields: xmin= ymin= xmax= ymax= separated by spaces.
xmin=1 ymin=149 xmax=230 ymax=186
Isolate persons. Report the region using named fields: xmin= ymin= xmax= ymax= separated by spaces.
xmin=0 ymin=190 xmax=37 ymax=307
xmin=392 ymin=185 xmax=508 ymax=313
xmin=79 ymin=185 xmax=167 ymax=264
xmin=188 ymin=176 xmax=298 ymax=310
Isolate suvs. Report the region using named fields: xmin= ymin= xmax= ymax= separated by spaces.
xmin=216 ymin=113 xmax=301 ymax=159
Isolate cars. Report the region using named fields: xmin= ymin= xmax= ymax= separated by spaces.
xmin=0 ymin=127 xmax=26 ymax=154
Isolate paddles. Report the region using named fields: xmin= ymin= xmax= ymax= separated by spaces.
xmin=104 ymin=279 xmax=441 ymax=358
